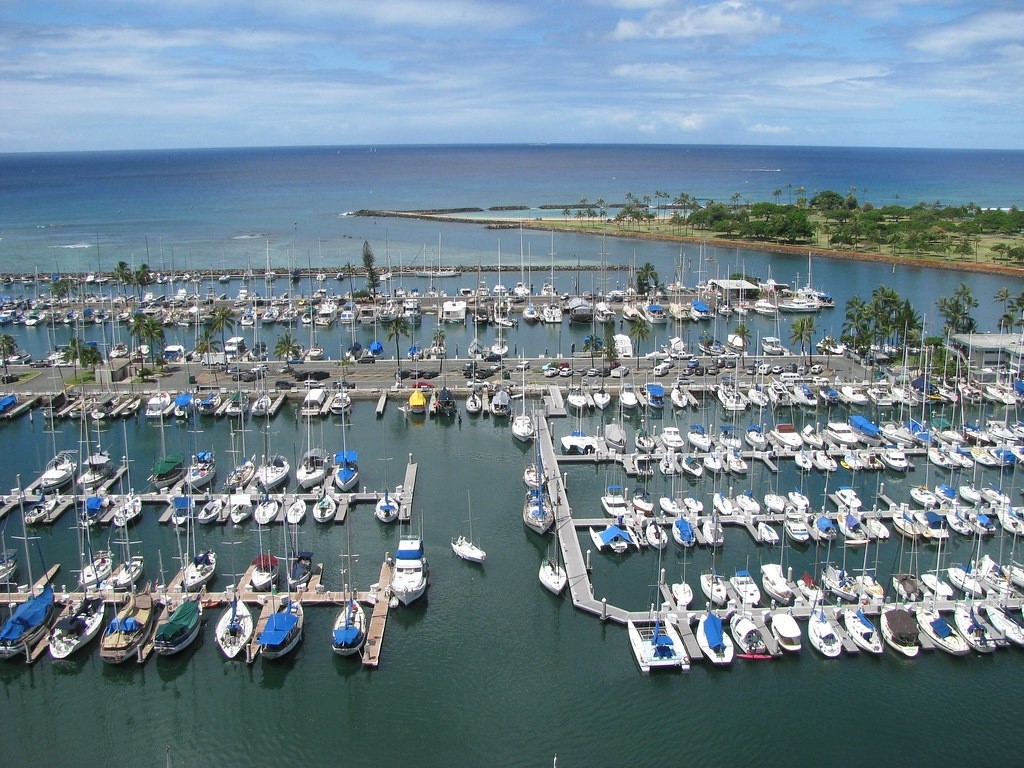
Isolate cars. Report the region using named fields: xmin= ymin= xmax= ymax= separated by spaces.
xmin=227 ymin=356 xmax=349 ymax=390
xmin=54 ymin=362 xmax=74 ymax=367
xmin=394 ymin=353 xmax=629 ymax=388
xmin=644 ymin=350 xmax=829 ymax=382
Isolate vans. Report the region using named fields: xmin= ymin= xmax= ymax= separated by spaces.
xmin=357 ymin=356 xmax=375 ymax=363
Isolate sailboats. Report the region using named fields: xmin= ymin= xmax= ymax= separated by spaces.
xmin=41 ymin=345 xmax=354 ymax=421
xmin=569 ymin=231 xmax=835 ymax=324
xmin=25 ymin=396 xmax=358 ymax=525
xmin=628 ymin=515 xmax=1024 ymax=674
xmin=562 ymin=391 xmax=1024 ymax=480
xmin=0 ymin=471 xmax=368 ymax=666
xmin=587 ymin=434 xmax=1024 ymax=558
xmin=567 ymin=313 xmax=1024 ymax=410
xmin=408 ymin=346 xmax=533 ymax=444
xmin=0 ymin=358 xmax=18 ymax=414
xmin=0 ymin=221 xmax=564 ymax=325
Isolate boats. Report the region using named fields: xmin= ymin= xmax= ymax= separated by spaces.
xmin=379 ymin=400 xmax=568 ymax=608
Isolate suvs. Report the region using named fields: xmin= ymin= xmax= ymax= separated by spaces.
xmin=2 ymin=374 xmax=19 ymax=384
xmin=30 ymin=360 xmax=51 ymax=368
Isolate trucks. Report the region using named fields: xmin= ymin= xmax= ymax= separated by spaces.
xmin=201 ymin=352 xmax=226 ymax=366
